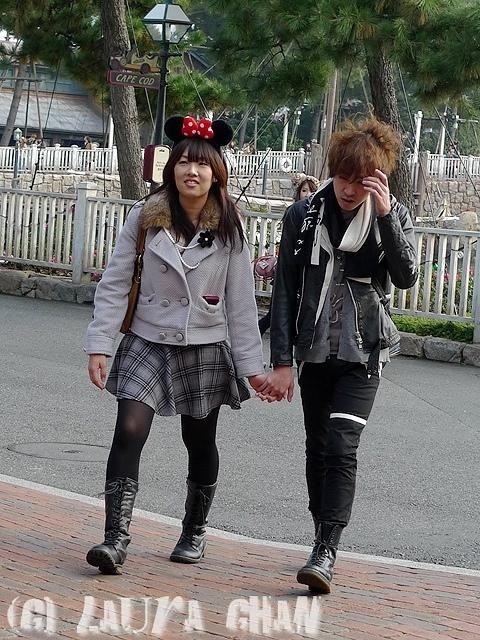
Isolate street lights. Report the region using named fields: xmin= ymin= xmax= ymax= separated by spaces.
xmin=144 ymin=2 xmax=192 ymax=197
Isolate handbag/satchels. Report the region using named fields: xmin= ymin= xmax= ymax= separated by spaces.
xmin=120 ymin=277 xmax=141 ymax=334
xmin=254 ymin=254 xmax=278 ymax=275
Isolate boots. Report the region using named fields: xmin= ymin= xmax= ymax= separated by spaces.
xmin=169 ymin=474 xmax=218 ymax=564
xmin=85 ymin=478 xmax=139 ymax=576
xmin=296 ymin=522 xmax=344 ymax=594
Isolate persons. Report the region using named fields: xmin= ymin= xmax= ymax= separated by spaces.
xmin=86 ymin=115 xmax=287 ymax=574
xmin=83 ymin=135 xmax=93 ymax=171
xmin=31 ymin=132 xmax=45 ymax=170
xmin=257 ymin=117 xmax=419 ymax=594
xmin=225 ymin=138 xmax=256 ymax=176
xmin=304 ymin=137 xmax=317 ymax=165
xmin=259 ymin=173 xmax=320 ymax=337
xmin=445 ymin=139 xmax=460 ymax=178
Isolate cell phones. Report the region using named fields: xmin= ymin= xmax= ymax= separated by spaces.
xmin=204 ymin=296 xmax=219 ymax=305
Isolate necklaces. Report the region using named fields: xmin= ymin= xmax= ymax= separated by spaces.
xmin=161 ymin=225 xmax=211 ymax=269
